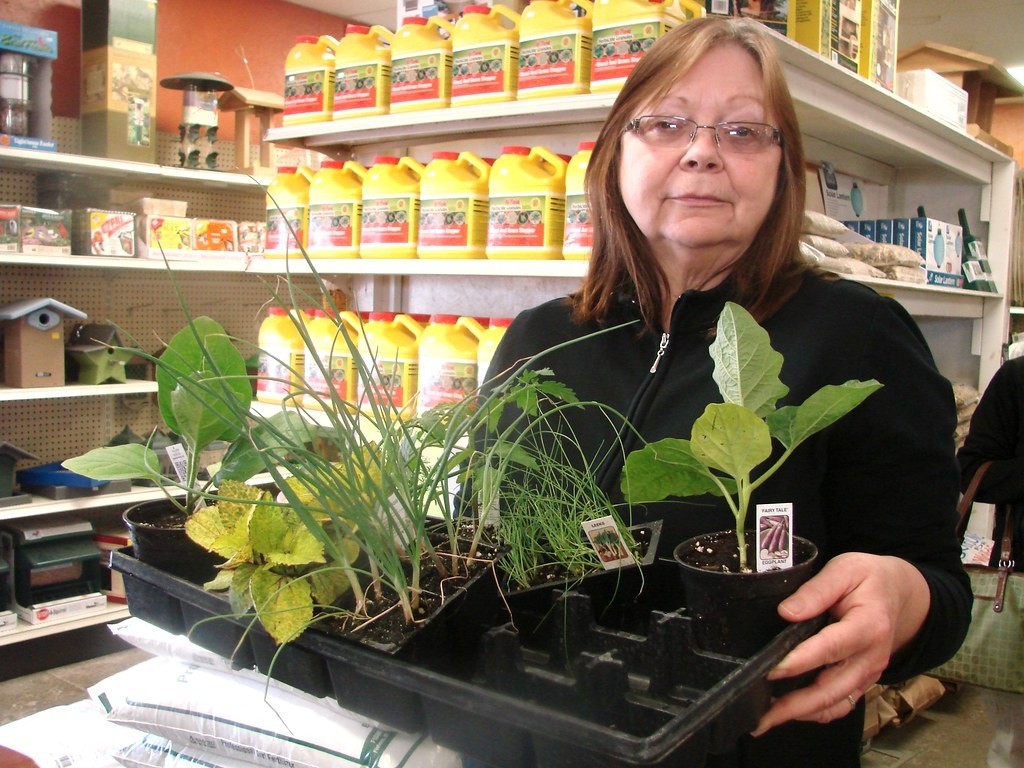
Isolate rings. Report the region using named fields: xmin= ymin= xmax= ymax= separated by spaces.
xmin=848 ymin=694 xmax=857 ymax=713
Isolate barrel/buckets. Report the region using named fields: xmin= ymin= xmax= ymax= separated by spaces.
xmin=282 ymin=35 xmax=337 ymax=126
xmin=562 ymin=142 xmax=601 ymax=260
xmin=363 ymin=142 xmax=572 ymax=259
xmin=516 ymin=0 xmax=592 ymax=98
xmin=450 ymin=4 xmax=520 ymax=105
xmin=389 ymin=16 xmax=453 ymax=114
xmin=302 ymin=160 xmax=365 ymax=259
xmin=265 ymin=167 xmax=310 ymax=258
xmin=589 ymin=1 xmax=707 ymax=93
xmin=256 ymin=306 xmax=518 ymax=434
xmin=331 ymin=25 xmax=393 ymax=120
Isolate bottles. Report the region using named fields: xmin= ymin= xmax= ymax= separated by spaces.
xmin=0 ymin=52 xmax=29 ymax=136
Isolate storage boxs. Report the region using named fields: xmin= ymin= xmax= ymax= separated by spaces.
xmin=81 ymin=0 xmax=156 ymax=163
xmin=3 ymin=314 xmax=65 ymax=388
xmin=844 ymin=217 xmax=964 ymax=286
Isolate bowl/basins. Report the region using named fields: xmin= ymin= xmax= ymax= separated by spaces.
xmin=183 ymin=439 xmax=230 ymax=469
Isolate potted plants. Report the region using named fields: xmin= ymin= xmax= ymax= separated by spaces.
xmin=62 ymin=194 xmax=883 ymax=663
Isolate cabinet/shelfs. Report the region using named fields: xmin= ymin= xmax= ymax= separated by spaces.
xmin=237 ymin=15 xmax=1015 ymax=522
xmin=0 ymin=144 xmax=271 ymax=641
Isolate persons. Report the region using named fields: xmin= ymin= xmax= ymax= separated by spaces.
xmin=956 ymin=355 xmax=1023 ymax=573
xmin=452 ymin=16 xmax=974 ymax=768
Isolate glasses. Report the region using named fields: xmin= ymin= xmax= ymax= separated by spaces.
xmin=618 ymin=115 xmax=782 ymax=155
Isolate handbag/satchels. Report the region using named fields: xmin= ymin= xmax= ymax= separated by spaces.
xmin=920 ymin=461 xmax=1024 ymax=693
